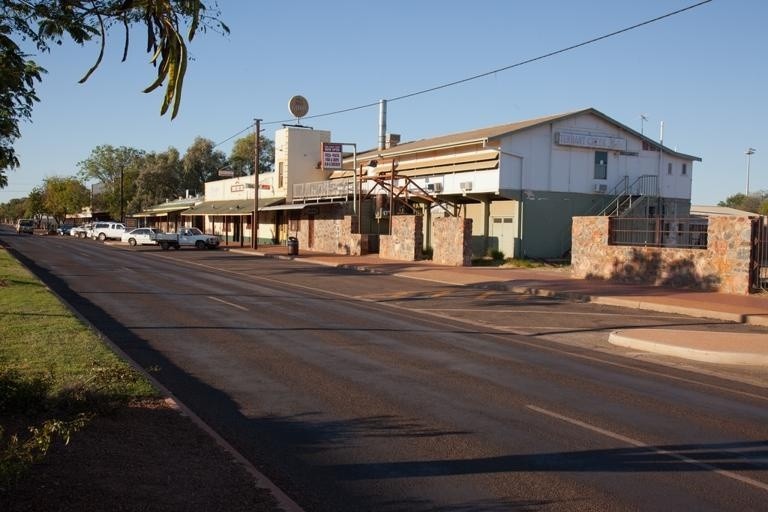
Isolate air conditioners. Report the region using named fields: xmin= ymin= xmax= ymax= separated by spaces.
xmin=593 ymin=183 xmax=609 ymax=194
xmin=426 ymin=183 xmax=441 ymax=192
xmin=459 ymin=182 xmax=472 ymax=191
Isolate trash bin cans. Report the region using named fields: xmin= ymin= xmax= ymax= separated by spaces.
xmin=288 ymin=237 xmax=298 ymax=255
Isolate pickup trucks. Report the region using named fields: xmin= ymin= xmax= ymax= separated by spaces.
xmin=155 ymin=227 xmax=220 ymax=250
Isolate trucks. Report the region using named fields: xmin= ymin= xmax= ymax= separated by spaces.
xmin=15 ymin=219 xmax=35 ymax=235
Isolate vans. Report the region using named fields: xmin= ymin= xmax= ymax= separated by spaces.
xmin=91 ymin=221 xmax=129 ymax=240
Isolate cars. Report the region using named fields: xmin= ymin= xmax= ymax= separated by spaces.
xmin=56 ymin=223 xmax=91 ymax=239
xmin=121 ymin=228 xmax=163 ymax=246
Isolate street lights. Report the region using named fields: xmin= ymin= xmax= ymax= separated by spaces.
xmin=744 ymin=148 xmax=757 ymax=195
